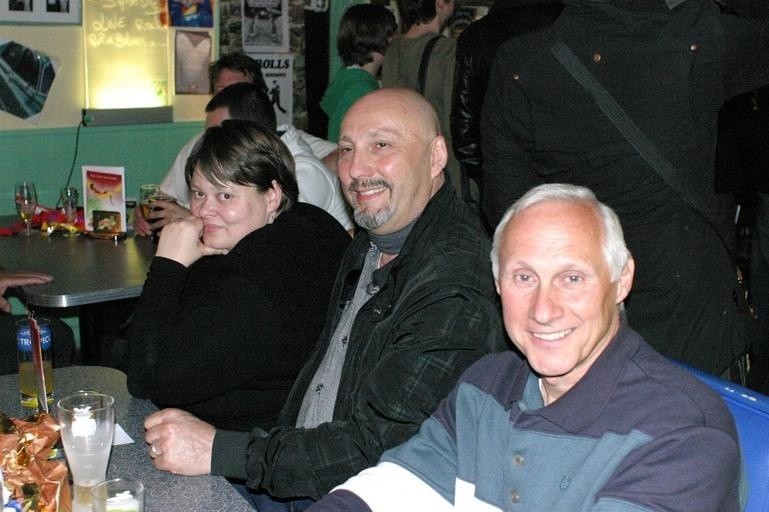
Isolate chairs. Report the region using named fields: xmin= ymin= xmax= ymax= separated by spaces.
xmin=669 ymin=360 xmax=769 ymax=510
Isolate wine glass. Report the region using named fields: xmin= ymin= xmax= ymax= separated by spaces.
xmin=60 ymin=185 xmax=84 ymax=238
xmin=13 ymin=181 xmax=41 ymax=236
xmin=140 ymin=182 xmax=170 ymax=247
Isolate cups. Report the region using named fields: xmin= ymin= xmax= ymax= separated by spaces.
xmin=15 ymin=313 xmax=60 ymax=407
xmin=57 ymin=391 xmax=115 ymax=491
xmin=146 ymin=194 xmax=178 ymax=245
xmin=91 ymin=477 xmax=148 ymax=511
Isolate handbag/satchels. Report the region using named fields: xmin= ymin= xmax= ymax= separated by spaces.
xmin=732 ymin=191 xmax=757 ymax=318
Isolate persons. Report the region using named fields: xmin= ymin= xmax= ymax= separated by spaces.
xmin=322 ymin=1 xmax=769 ymax=391
xmin=124 ymin=51 xmax=357 ymax=426
xmin=0 ymin=270 xmax=55 ymax=312
xmin=295 ymin=184 xmax=742 ymax=512
xmin=145 ymin=85 xmax=515 ymax=511
